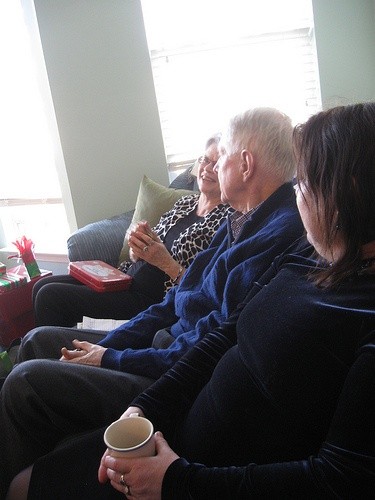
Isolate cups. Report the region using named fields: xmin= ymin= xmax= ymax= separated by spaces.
xmin=103 ymin=413 xmax=157 ymax=457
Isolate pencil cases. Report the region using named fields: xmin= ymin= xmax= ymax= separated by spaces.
xmin=68 ymin=260 xmax=133 ymax=293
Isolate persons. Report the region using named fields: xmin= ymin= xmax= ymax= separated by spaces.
xmin=8 ymin=102 xmax=375 ymax=500
xmin=32 ymin=135 xmax=236 ymax=327
xmin=0 ymin=108 xmax=331 ymax=498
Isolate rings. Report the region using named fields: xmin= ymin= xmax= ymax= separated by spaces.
xmin=124 ymin=486 xmax=131 ymax=496
xmin=120 ymin=473 xmax=128 ymax=487
xmin=149 ymin=241 xmax=155 ymax=246
xmin=143 ymin=246 xmax=149 ymax=251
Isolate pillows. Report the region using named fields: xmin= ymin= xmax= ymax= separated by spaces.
xmin=116 ymin=175 xmax=198 ymax=272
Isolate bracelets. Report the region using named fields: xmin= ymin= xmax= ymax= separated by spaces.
xmin=173 ymin=267 xmax=185 ymax=283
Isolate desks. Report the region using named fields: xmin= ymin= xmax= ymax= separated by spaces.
xmin=0 ymin=261 xmax=53 ymax=349
